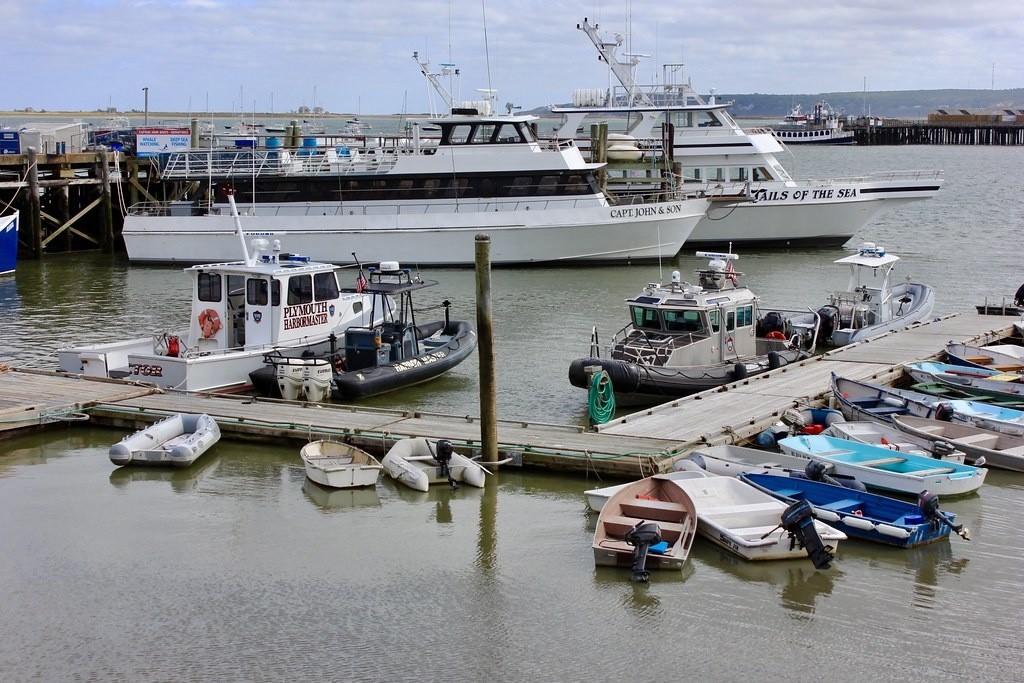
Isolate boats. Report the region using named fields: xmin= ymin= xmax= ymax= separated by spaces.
xmin=750 ymin=101 xmax=855 ymax=144
xmin=249 ymin=317 xmax=478 ymax=403
xmin=581 ymin=320 xmax=1023 ymax=583
xmin=422 ymin=124 xmax=440 ymax=131
xmin=121 ymin=52 xmax=756 ymax=268
xmin=381 ymin=435 xmax=488 ymax=491
xmin=541 ymin=18 xmax=946 ymax=249
xmin=105 ymin=413 xmax=223 ymax=470
xmin=300 ymin=439 xmax=382 ymax=491
xmin=96 ymin=117 xmax=133 ymax=131
xmin=568 ymin=250 xmax=820 ymax=408
xmin=784 ymin=244 xmax=937 ymax=346
xmin=54 ymin=239 xmax=396 ymax=395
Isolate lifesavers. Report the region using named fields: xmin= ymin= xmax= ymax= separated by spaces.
xmin=199 ymin=309 xmax=220 ymax=334
xmin=765 ymin=331 xmax=786 ymax=340
xmin=222 ymin=183 xmax=239 ymax=198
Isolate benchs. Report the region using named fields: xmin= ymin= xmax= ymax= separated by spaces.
xmin=879 ymin=442 xmax=916 ymax=453
xmin=697 ymin=501 xmax=785 ymax=528
xmin=918 ymin=425 xmax=945 ymax=436
xmin=603 ymin=514 xmax=684 ymax=546
xmin=621 ymin=498 xmax=687 ymax=523
xmin=854 ymin=456 xmax=906 ymax=466
xmin=775 ymin=488 xmax=804 ymax=500
xmin=849 ymin=429 xmax=884 ymax=443
xmin=955 ymin=432 xmax=1000 ymax=449
xmin=308 ymin=454 xmax=434 ymax=467
xmin=847 ymin=396 xmax=884 ymax=408
xmin=865 ymin=406 xmax=910 ymax=416
xmin=816 ymin=448 xmax=853 ymax=461
xmin=822 ymin=498 xmax=864 ymax=513
xmin=731 ymin=523 xmax=826 ymax=542
xmin=1001 ymin=445 xmax=1024 ymax=456
xmin=893 ymin=512 xmax=919 ymax=524
xmin=906 ymin=467 xmax=955 ymax=476
xmin=927 ymin=355 xmax=1024 ymax=405
xmin=757 ymin=461 xmax=781 ymax=467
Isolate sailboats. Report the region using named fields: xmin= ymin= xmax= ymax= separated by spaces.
xmin=163 ymin=84 xmax=372 ymax=134
xmin=397 ymin=89 xmax=411 ymax=133
